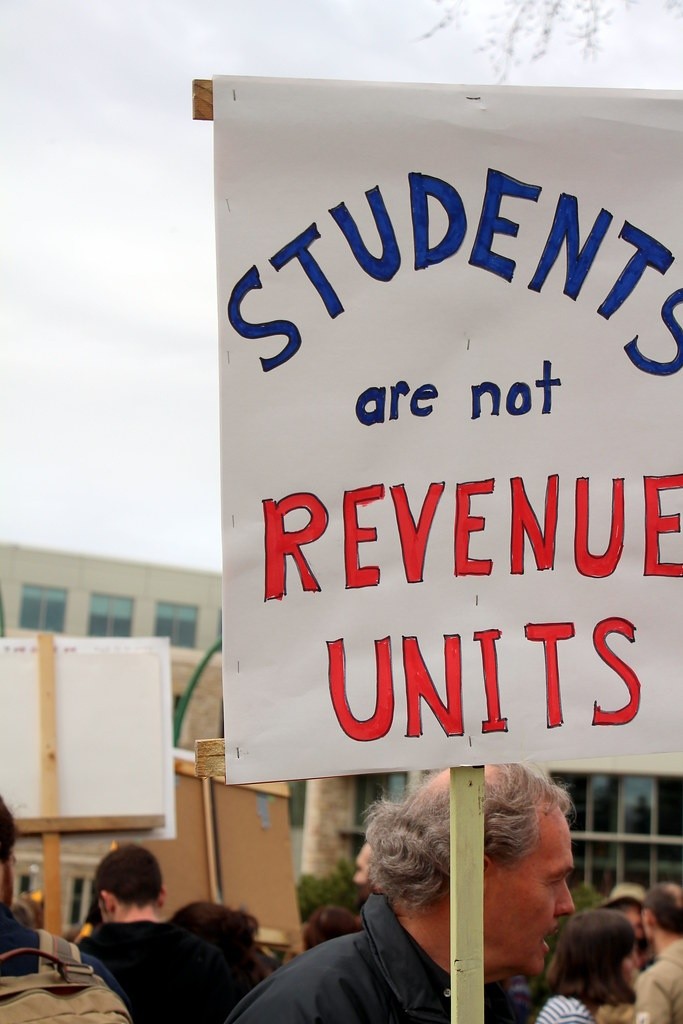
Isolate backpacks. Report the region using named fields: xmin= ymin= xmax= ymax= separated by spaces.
xmin=0 ymin=929 xmax=133 ymax=1024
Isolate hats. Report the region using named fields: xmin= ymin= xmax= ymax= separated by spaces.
xmin=599 ymin=881 xmax=648 ymax=909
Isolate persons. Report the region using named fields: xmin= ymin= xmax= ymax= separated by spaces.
xmin=0 ymin=763 xmax=683 ymax=1023
